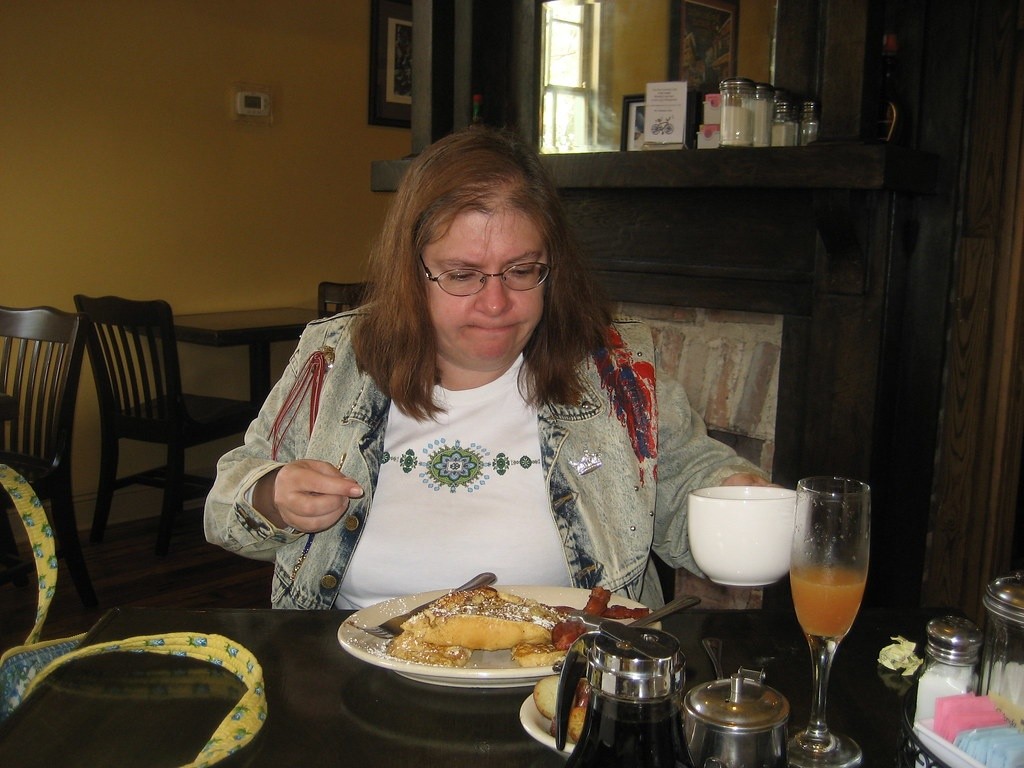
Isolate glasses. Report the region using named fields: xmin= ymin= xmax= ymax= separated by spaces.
xmin=420 ymin=257 xmax=551 ymax=297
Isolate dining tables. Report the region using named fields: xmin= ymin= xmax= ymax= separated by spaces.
xmin=0 ymin=601 xmax=1024 ymax=768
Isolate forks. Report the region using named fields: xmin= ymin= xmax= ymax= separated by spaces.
xmin=347 ymin=572 xmax=496 ymax=639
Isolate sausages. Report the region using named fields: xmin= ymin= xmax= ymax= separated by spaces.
xmin=583 ymin=585 xmax=611 ymax=615
xmin=552 ymin=620 xmax=585 ymax=650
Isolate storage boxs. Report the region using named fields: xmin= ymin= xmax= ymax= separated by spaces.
xmin=703 ymin=94 xmax=721 ymax=125
xmin=695 ymin=124 xmax=720 ymax=149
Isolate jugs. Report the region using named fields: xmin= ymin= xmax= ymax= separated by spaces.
xmin=555 ymin=620 xmax=695 ymax=768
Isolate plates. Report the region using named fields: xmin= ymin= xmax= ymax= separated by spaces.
xmin=337 ymin=585 xmax=661 ymax=687
xmin=520 ymin=692 xmax=576 ymax=761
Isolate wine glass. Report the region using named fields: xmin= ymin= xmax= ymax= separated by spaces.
xmin=791 ymin=475 xmax=870 ymax=768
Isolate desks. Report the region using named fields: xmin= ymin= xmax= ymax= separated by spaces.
xmin=124 ymin=307 xmax=343 ymax=404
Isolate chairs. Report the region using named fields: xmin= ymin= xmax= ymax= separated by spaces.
xmin=318 ymin=281 xmax=373 ymax=312
xmin=0 ymin=305 xmax=100 ymax=611
xmin=72 ymin=294 xmax=263 ymax=557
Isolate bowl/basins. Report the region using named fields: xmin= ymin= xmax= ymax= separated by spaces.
xmin=688 ymin=486 xmax=797 ymax=586
xmin=684 ymin=668 xmax=789 ymax=768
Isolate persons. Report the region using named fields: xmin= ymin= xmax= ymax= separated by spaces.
xmin=203 ymin=126 xmax=782 ymax=610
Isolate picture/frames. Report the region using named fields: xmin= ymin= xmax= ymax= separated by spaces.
xmin=670 ymin=0 xmax=741 ymax=94
xmin=366 ymin=0 xmax=411 ymax=128
xmin=620 ymin=91 xmax=698 ymax=150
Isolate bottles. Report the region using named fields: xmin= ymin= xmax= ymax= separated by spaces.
xmin=800 ymin=102 xmax=818 ymax=146
xmin=980 ymin=572 xmax=1024 ymax=705
xmin=772 ymin=102 xmax=798 ymax=146
xmin=719 ymin=78 xmax=755 ymax=147
xmin=754 ymin=82 xmax=775 ymax=147
xmin=912 ymin=616 xmax=983 ymax=723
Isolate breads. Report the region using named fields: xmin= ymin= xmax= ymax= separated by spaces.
xmin=399 ymin=591 xmax=563 ymax=652
xmin=534 ymin=674 xmax=590 ymax=742
